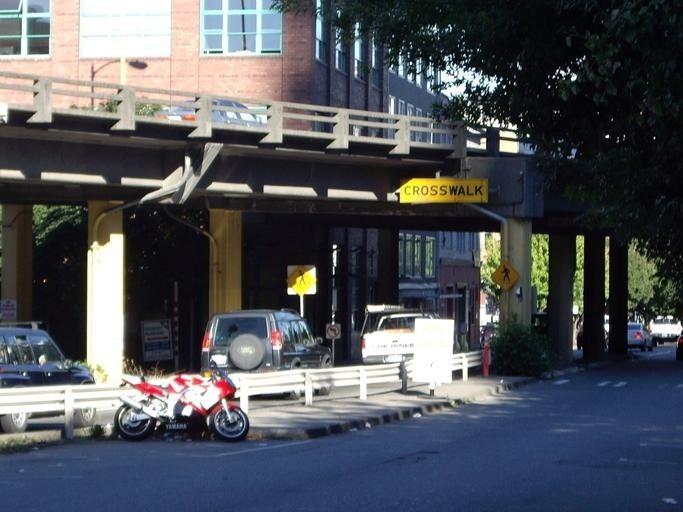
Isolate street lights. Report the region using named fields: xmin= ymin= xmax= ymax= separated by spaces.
xmin=90 ymin=57 xmax=147 ymax=107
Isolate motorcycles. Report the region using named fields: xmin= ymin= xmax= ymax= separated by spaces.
xmin=111 ymin=359 xmax=247 ymax=443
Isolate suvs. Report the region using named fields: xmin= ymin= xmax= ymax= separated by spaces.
xmin=0 ymin=320 xmax=97 ymax=432
xmin=197 ymin=306 xmax=335 ymax=399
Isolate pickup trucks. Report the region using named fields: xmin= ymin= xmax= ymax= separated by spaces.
xmin=356 ymin=310 xmax=443 ymax=365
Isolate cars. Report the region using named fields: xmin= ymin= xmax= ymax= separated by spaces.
xmin=157 ymin=101 xmax=265 ymax=129
xmin=573 ymin=311 xmax=682 ymax=361
